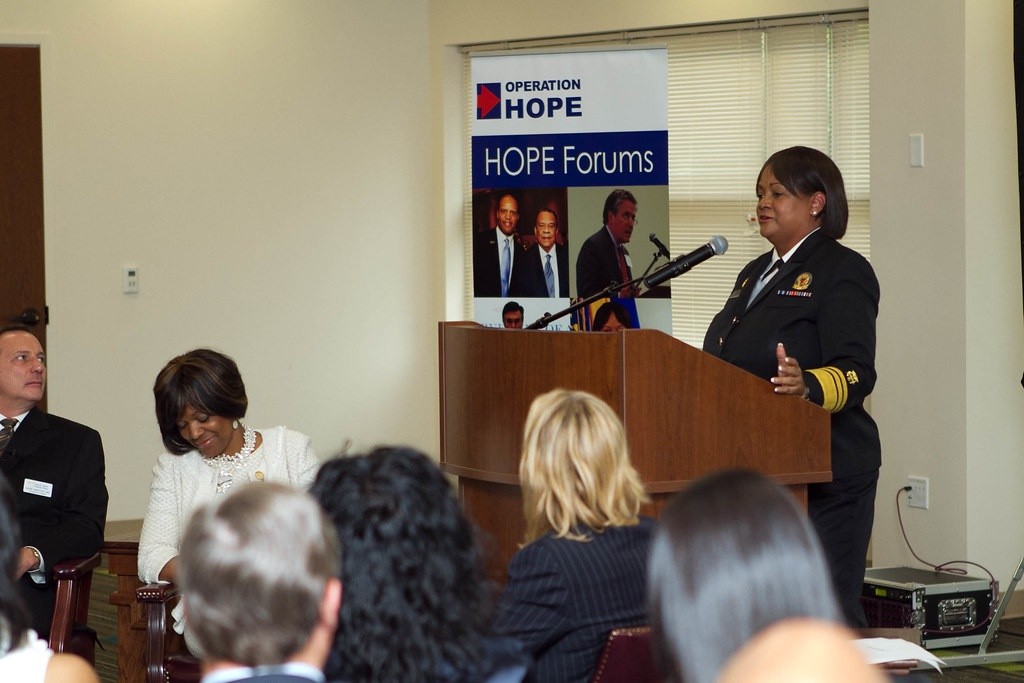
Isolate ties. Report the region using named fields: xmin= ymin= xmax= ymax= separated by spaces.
xmin=617 ymin=243 xmax=629 ymax=282
xmin=0 ymin=418 xmax=19 ymax=458
xmin=501 ymin=238 xmax=511 ymax=298
xmin=545 ymin=254 xmax=555 ymax=298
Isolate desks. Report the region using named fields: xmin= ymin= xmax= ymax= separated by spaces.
xmin=104 ymin=531 xmax=148 ymax=683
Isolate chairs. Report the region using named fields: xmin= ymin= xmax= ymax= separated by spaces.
xmin=49 ymin=552 xmax=101 ymax=672
xmin=596 ymin=627 xmax=655 ymax=683
xmin=136 ymin=583 xmax=200 ymax=683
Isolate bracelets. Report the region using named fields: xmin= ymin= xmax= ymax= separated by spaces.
xmin=29 ymin=547 xmax=40 ymax=559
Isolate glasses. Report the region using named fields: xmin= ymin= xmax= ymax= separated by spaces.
xmin=618 ymin=210 xmax=638 ymax=225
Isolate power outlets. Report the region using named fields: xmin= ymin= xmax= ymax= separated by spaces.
xmin=907 ymin=477 xmax=929 ymax=510
xmin=124 ymin=267 xmax=139 ymax=293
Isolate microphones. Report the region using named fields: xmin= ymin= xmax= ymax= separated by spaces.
xmin=649 ymin=233 xmax=670 ymax=260
xmin=643 ymin=235 xmax=729 ymax=289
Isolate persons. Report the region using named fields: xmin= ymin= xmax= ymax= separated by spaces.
xmin=502 ymin=301 xmax=524 ymax=329
xmin=0 ymin=561 xmax=100 ymax=683
xmin=138 ymin=348 xmax=321 ymax=588
xmin=309 ymin=446 xmax=507 ymax=683
xmin=0 ymin=326 xmax=109 ymax=659
xmin=525 ymin=208 xmax=570 ymax=298
xmin=472 ymin=193 xmax=526 ymax=297
xmin=576 ymin=189 xmax=640 ymax=331
xmin=702 ymin=146 xmax=882 ymax=627
xmin=496 ymin=388 xmax=663 ymax=683
xmin=647 ymin=465 xmax=848 ymax=683
xmin=592 ymin=302 xmax=632 ymax=332
xmin=175 ymin=482 xmax=344 ymax=683
